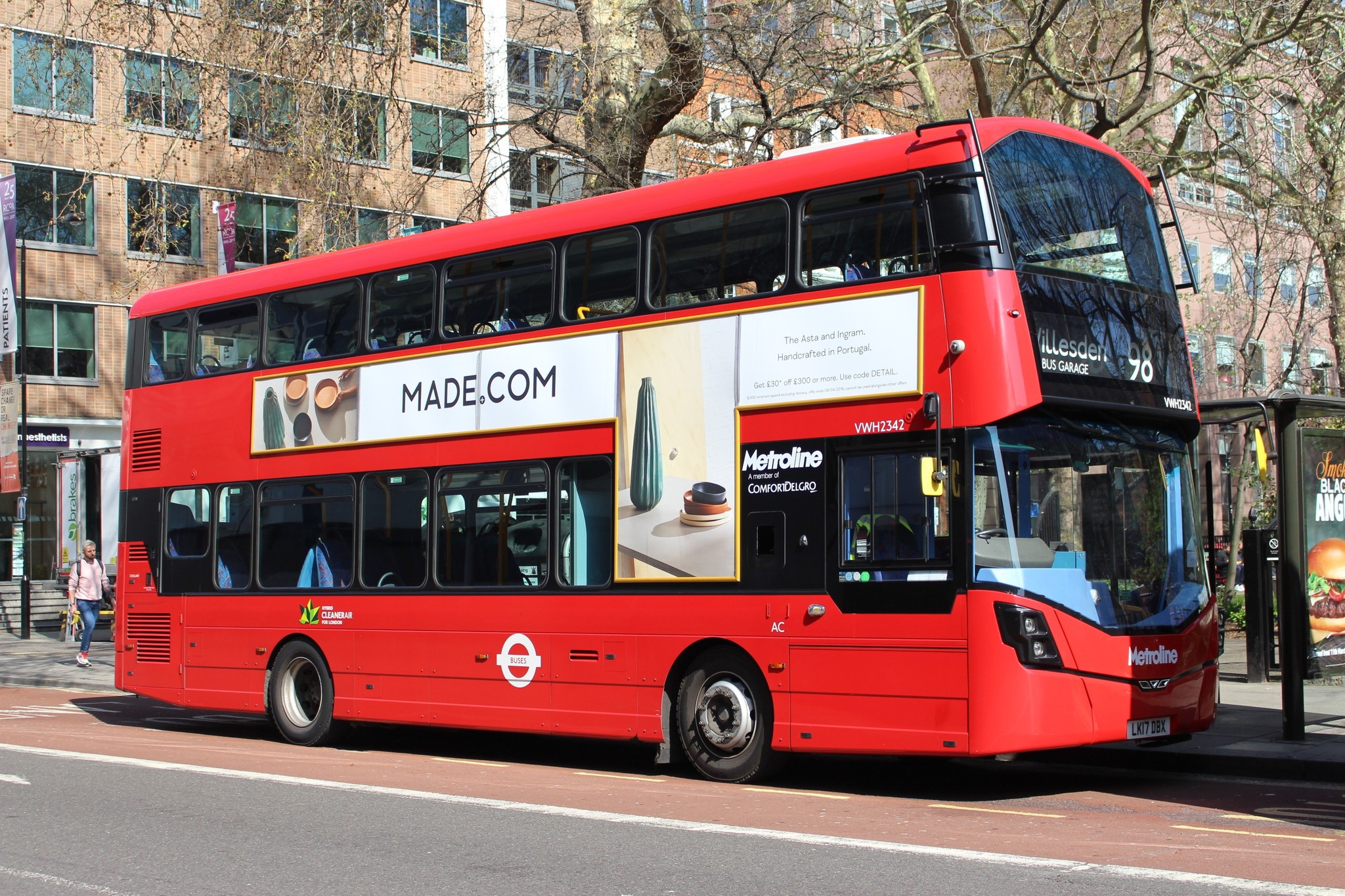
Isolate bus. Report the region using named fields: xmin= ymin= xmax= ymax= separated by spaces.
xmin=115 ymin=108 xmax=1278 ymax=783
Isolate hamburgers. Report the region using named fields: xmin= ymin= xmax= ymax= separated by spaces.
xmin=1307 ymin=537 xmax=1345 ymax=633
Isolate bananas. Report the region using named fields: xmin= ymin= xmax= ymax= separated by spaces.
xmin=71 ymin=614 xmax=78 ymax=625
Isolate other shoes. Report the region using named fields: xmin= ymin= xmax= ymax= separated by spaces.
xmin=1234 ymin=584 xmax=1245 ymax=592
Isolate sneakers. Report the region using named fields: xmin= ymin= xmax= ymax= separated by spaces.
xmin=77 ymin=659 xmax=92 ymax=667
xmin=76 ymin=653 xmax=88 ymax=665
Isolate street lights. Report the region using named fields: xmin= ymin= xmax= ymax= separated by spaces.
xmin=1215 ymin=424 xmax=1238 ymax=542
xmin=22 ymin=212 xmax=88 ymax=639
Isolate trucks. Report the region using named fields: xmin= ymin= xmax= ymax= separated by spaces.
xmin=55 ymin=448 xmax=227 ymax=643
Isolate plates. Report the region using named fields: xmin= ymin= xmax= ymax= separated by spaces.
xmin=679 ymin=507 xmax=732 ymax=527
xmin=283 ymin=377 xmax=308 ymax=406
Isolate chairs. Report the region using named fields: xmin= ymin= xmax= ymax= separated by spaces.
xmin=148 ymin=257 xmax=912 ymax=385
xmin=169 ymin=519 xmax=527 ymax=590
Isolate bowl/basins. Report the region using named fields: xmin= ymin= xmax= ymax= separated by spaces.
xmin=293 ymin=412 xmax=312 ymax=443
xmin=684 ymin=498 xmax=728 ymax=514
xmin=683 ymin=490 xmax=719 ymax=507
xmin=692 ymin=482 xmax=726 ymax=505
xmin=285 ymin=374 xmax=308 ymax=402
xmin=314 ymin=378 xmax=339 ymax=411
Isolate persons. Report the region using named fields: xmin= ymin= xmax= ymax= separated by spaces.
xmin=67 ymin=540 xmax=116 ymax=668
xmin=1215 ymin=540 xmax=1246 ymax=592
xmin=328 ymin=369 xmax=358 ymax=412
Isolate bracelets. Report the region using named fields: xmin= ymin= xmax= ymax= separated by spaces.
xmin=70 ymin=603 xmax=75 ymax=606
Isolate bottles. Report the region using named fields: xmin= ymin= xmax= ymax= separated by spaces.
xmin=630 ymin=377 xmax=663 ymax=511
xmin=263 ymin=387 xmax=285 ymax=450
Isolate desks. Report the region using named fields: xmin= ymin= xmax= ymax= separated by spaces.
xmin=618 ymin=475 xmax=735 ymax=578
